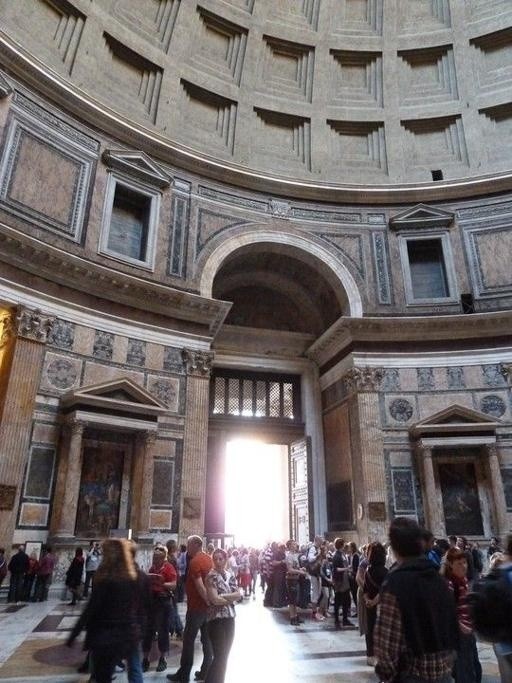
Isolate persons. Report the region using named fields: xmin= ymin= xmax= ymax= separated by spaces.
xmin=66 ymin=535 xmax=242 ymax=683
xmin=238 ymin=536 xmax=360 ymax=628
xmin=1 ymin=544 xmax=56 ymax=603
xmin=353 ymin=516 xmax=512 ymax=682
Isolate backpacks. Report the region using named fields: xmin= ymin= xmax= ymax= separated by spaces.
xmin=470 ymin=563 xmax=512 ymax=644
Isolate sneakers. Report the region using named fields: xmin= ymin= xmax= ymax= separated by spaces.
xmin=79 ymin=658 xmax=207 ymax=683
xmin=289 ymin=613 xmax=355 ymax=628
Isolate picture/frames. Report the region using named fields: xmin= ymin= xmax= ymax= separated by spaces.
xmin=25 ymin=541 xmax=43 ymax=562
